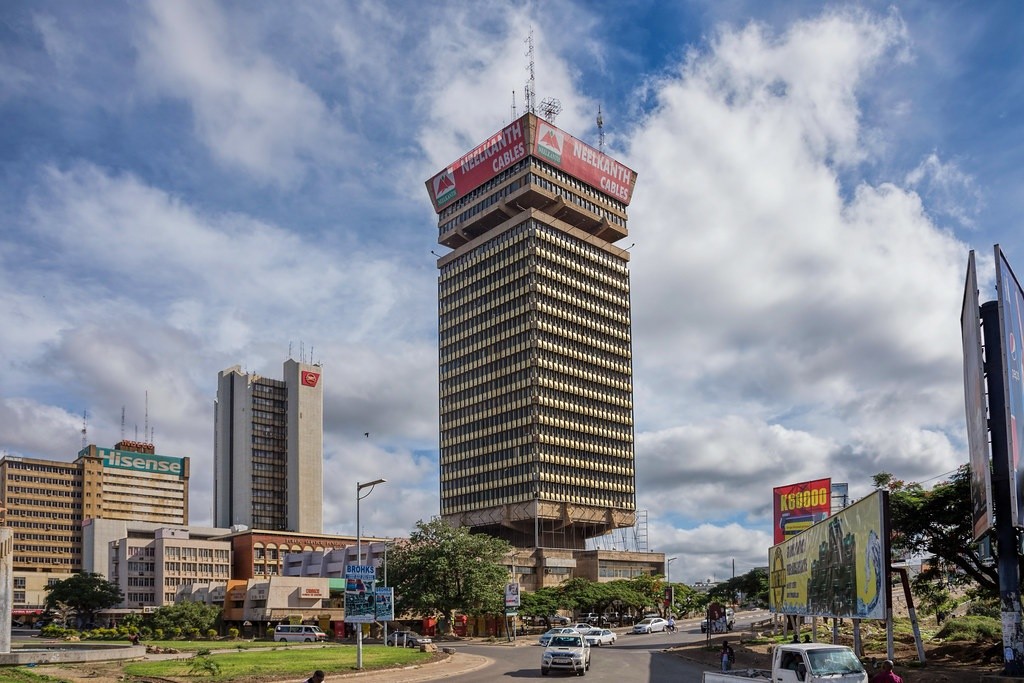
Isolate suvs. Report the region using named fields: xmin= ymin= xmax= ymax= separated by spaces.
xmin=608 ymin=613 xmax=632 ymax=623
xmin=701 ymin=608 xmax=736 ymax=633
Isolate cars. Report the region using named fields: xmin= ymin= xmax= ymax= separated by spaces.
xmin=644 ymin=609 xmax=678 ymax=621
xmin=633 ymin=618 xmax=669 ymax=634
xmin=534 ymin=614 xmax=571 ymax=625
xmin=577 ymin=613 xmax=607 ymax=625
xmin=35 ymin=621 xmax=46 ymax=628
xmin=12 ymin=619 xmax=23 ymax=627
xmin=388 ymin=631 xmax=431 ymax=648
xmin=568 ymin=623 xmax=601 ymax=635
xmin=584 ymin=629 xmax=617 ymax=646
xmin=54 ymin=622 xmax=101 ymax=630
xmin=539 ymin=627 xmax=580 ymax=644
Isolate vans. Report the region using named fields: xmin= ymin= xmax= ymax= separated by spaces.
xmin=274 ymin=625 xmax=329 ymax=642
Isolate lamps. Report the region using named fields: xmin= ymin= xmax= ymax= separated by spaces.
xmin=624 ymin=548 xmax=628 ymax=551
xmin=613 ymin=547 xmax=616 ymax=551
xmin=597 ymin=545 xmax=600 ymax=549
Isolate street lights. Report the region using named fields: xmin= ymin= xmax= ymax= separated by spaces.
xmin=357 ymin=480 xmax=387 ymax=668
xmin=668 ymin=557 xmax=676 ymax=620
xmin=513 ymin=552 xmax=522 ymax=640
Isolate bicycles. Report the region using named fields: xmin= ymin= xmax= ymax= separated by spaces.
xmin=666 ymin=625 xmax=679 ymax=635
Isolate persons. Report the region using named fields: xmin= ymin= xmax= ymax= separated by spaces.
xmin=790 ymin=635 xmax=801 ymax=643
xmin=667 ymin=618 xmax=676 ymax=631
xmin=519 ymin=625 xmax=525 ymax=636
xmin=715 ymin=641 xmax=735 ymax=670
xmin=870 ymin=660 xmax=903 ymax=683
xmin=789 ymin=654 xmax=802 ymax=670
xmin=803 ymin=635 xmax=813 ymax=643
xmin=305 ymin=670 xmax=325 ymax=683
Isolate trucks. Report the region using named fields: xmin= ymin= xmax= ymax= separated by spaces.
xmin=703 ymin=641 xmax=868 ymax=683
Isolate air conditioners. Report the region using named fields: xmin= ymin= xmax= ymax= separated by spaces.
xmin=47 ymin=527 xmax=51 ymax=530
xmin=22 ymin=537 xmax=25 ymax=540
xmin=16 ymin=478 xmax=20 ymax=482
xmin=22 ymin=514 xmax=26 ymax=517
xmin=22 ymin=549 xmax=25 ymax=551
xmin=54 ymin=550 xmax=57 ymax=553
xmin=545 ymin=568 xmax=552 ymax=573
xmin=28 ymin=561 xmax=31 ymax=563
xmin=60 ymin=551 xmax=63 ymax=553
xmin=53 ymin=562 xmax=60 ymax=564
xmin=16 ymin=502 xmax=20 ymax=505
